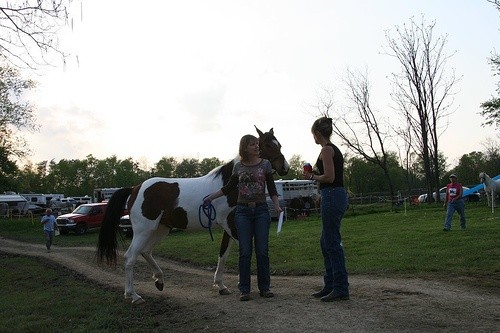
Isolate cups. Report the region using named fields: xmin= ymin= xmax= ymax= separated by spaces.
xmin=304 ymin=164 xmax=312 ymax=180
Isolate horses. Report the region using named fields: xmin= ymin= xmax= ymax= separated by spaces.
xmin=478 ymin=172 xmax=500 ymax=214
xmin=92 ymin=124 xmax=290 ymax=305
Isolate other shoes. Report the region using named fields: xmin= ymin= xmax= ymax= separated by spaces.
xmin=311 ymin=275 xmax=334 ymax=298
xmin=239 ymin=290 xmax=252 ymax=302
xmin=320 ymin=280 xmax=350 ymax=302
xmin=260 ymin=288 xmax=274 ymax=297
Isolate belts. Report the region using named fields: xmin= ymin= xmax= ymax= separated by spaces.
xmin=237 ymin=202 xmax=268 ymax=208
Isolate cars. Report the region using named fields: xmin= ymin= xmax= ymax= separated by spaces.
xmin=418 ymin=186 xmax=481 ymax=203
xmin=55 ymin=202 xmax=129 ymax=235
xmin=118 ymin=215 xmax=133 ymax=233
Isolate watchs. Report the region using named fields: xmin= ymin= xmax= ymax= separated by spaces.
xmin=309 ymin=174 xmax=314 ymax=180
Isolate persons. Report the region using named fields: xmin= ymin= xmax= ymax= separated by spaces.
xmin=443 ymin=174 xmax=467 ymax=232
xmin=302 ymin=117 xmax=350 ymax=301
xmin=41 ymin=208 xmax=59 ymax=252
xmin=203 ymin=135 xmax=283 ymax=301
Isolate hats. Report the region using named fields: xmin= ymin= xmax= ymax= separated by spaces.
xmin=448 ymin=174 xmax=457 ymax=178
